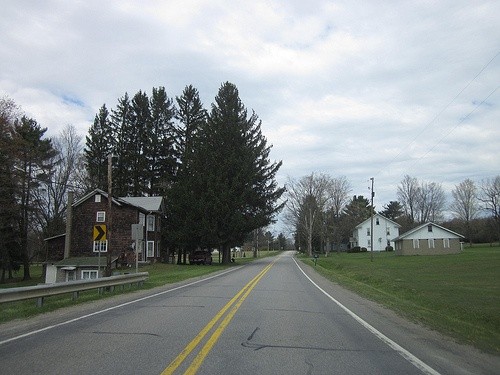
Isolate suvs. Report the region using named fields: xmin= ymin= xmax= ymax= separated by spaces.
xmin=190 ymin=250 xmax=212 ymax=264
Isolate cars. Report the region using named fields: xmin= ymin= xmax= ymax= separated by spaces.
xmin=230 ymin=245 xmax=242 ymax=252
